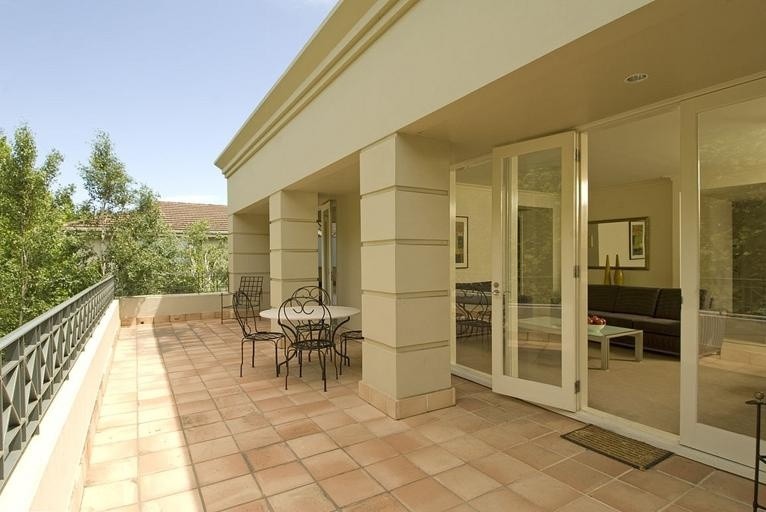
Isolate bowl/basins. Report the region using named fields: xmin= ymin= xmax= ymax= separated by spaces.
xmin=587 ymin=324 xmax=606 ymax=331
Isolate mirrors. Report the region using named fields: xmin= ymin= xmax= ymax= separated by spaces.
xmin=588 ymin=216 xmax=650 ymax=271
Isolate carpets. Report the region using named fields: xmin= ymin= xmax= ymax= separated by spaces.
xmin=559 ymin=423 xmax=674 ymax=471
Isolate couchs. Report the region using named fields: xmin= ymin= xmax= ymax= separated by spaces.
xmin=551 ymin=284 xmax=714 ymax=357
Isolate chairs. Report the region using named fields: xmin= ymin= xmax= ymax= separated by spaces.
xmin=220 ymin=276 xmax=364 ymax=392
xmin=455 ymin=285 xmax=491 ymax=344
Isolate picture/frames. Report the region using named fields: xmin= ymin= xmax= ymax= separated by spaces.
xmin=456 ymin=215 xmax=469 ymax=270
xmin=629 ymin=221 xmax=645 ymax=260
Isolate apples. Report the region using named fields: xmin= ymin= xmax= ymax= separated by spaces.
xmin=588 ymin=315 xmax=605 ymax=325
